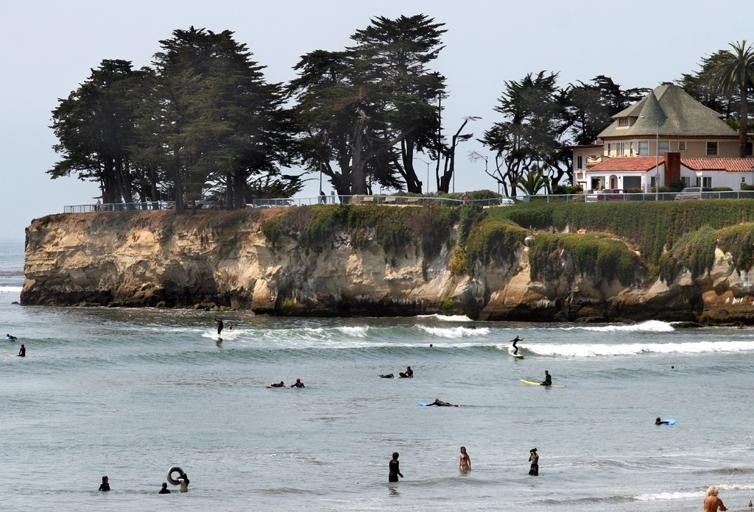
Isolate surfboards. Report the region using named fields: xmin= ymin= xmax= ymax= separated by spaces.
xmin=417 ymin=399 xmax=431 ymax=406
xmin=514 ymin=353 xmax=524 ymax=358
xmin=660 ymin=416 xmax=677 ymax=425
xmin=520 ymin=378 xmax=565 ymax=388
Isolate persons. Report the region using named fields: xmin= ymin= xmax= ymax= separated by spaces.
xmin=290 ymin=377 xmax=305 ymax=388
xmin=266 ymin=380 xmax=287 ymax=388
xmin=399 ymin=372 xmax=407 ymax=378
xmin=6 ymin=333 xmax=17 ymax=341
xmin=426 ymin=397 xmax=458 ymax=407
xmin=654 ymin=415 xmax=669 ymax=425
xmin=158 ymin=482 xmax=170 ymax=495
xmin=377 ymin=371 xmax=395 ymax=379
xmin=458 ymin=446 xmax=472 ymax=471
xmin=176 ymin=474 xmax=189 ymax=493
xmin=15 ymin=344 xmax=26 ymax=358
xmin=527 ymin=448 xmax=539 ymax=476
xmin=403 ymin=365 xmax=414 ymax=378
xmin=703 ymin=485 xmax=727 ymax=512
xmin=388 ymin=452 xmax=404 ymax=482
xmin=98 ymin=475 xmax=110 ymax=491
xmin=509 ymin=334 xmax=525 ymax=354
xmin=214 ymin=317 xmax=224 ymax=334
xmin=539 ymin=369 xmax=551 ymax=387
xmin=319 ymin=191 xmax=326 ymax=204
xmin=229 ymin=325 xmax=233 ymax=330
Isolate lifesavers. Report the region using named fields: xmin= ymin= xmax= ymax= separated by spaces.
xmin=167 ymin=466 xmax=184 ymax=485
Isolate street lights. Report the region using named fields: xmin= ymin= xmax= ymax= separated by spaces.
xmin=639 ymin=115 xmax=659 ymax=202
